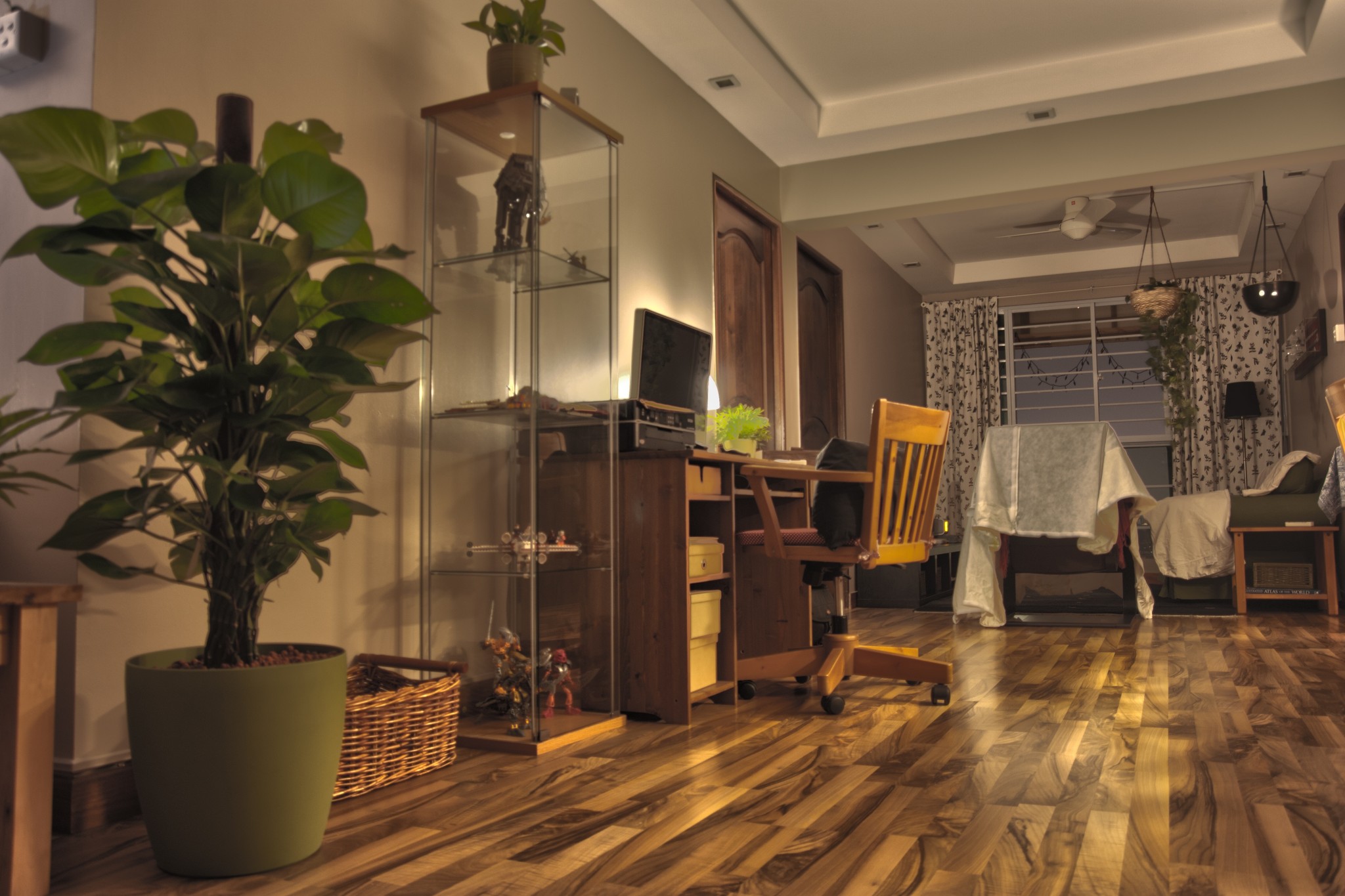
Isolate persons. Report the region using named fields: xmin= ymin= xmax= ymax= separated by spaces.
xmin=540 ymin=650 xmax=581 ymax=718
xmin=508 ymin=525 xmax=566 ymax=547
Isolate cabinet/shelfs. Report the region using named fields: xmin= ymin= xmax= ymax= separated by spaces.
xmin=1279 ymin=309 xmax=1331 ymax=384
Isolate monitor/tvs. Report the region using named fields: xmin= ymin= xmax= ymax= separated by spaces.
xmin=629 ymin=308 xmax=713 ymax=448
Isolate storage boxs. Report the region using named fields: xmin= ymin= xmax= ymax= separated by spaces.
xmin=412 ymin=84 xmax=635 ymax=765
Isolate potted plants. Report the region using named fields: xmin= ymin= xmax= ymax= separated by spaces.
xmin=0 ymin=79 xmax=444 ymax=883
xmin=699 ymin=400 xmax=777 ymax=462
xmin=1118 ymin=180 xmax=1206 ymax=438
xmin=457 ymin=0 xmax=576 ymax=100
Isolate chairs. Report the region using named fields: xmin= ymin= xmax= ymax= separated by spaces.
xmin=951 ymin=418 xmax=1158 ymax=635
xmin=729 ymin=395 xmax=956 ymax=714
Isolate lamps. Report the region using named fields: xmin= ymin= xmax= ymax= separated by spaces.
xmin=1221 ymin=382 xmax=1259 ymax=496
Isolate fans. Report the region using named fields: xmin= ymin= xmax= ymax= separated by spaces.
xmin=988 ymin=196 xmax=1149 ymax=243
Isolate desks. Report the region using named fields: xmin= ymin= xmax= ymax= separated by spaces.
xmin=1221 ymin=521 xmax=1340 ymax=621
xmin=550 ymin=447 xmax=864 ymax=732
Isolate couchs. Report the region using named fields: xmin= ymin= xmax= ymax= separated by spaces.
xmin=1144 ymin=445 xmax=1336 ymax=609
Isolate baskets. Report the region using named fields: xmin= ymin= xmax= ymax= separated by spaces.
xmin=335 ymin=650 xmax=467 ymax=805
